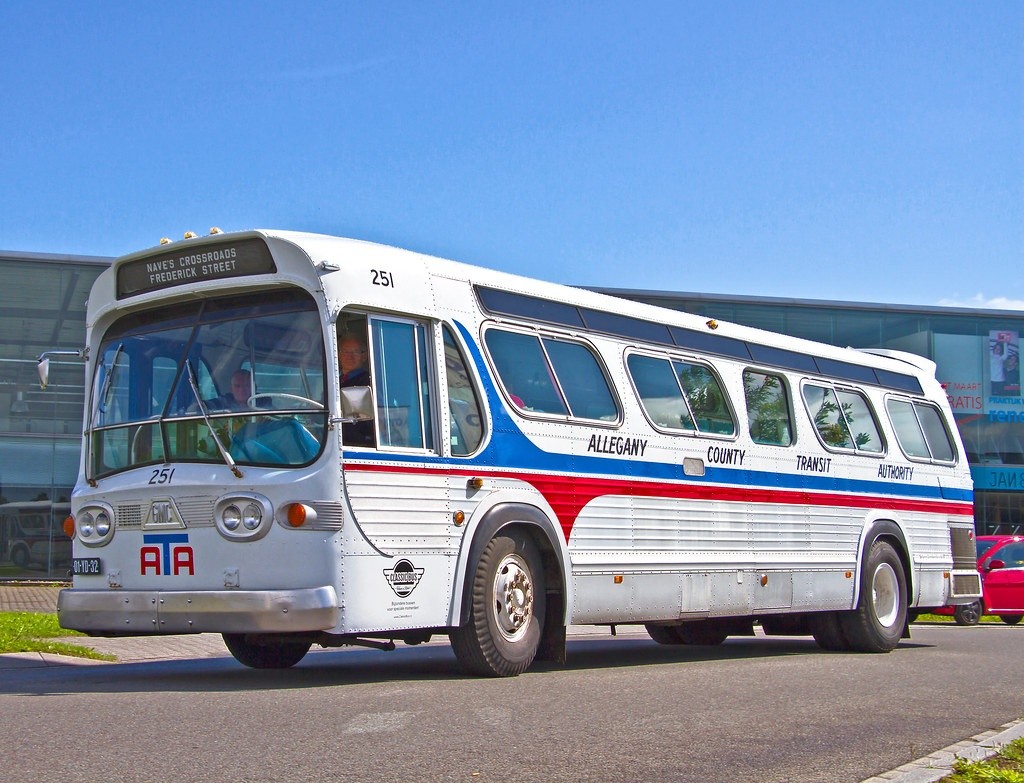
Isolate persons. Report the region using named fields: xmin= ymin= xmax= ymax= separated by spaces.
xmin=296 ymin=335 xmax=385 ymax=448
xmin=990 ymin=335 xmax=1008 ymax=395
xmin=187 ymin=369 xmax=273 ymax=432
xmin=1003 ymin=355 xmax=1019 ymax=395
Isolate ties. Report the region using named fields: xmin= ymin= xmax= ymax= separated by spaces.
xmin=339 ymin=373 xmax=348 ymax=387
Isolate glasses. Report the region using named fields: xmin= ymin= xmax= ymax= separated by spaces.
xmin=340 ymin=349 xmax=368 ymax=356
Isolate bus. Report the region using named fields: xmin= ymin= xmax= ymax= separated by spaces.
xmin=35 ymin=226 xmax=984 ymax=679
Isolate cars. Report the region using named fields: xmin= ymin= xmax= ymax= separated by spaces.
xmin=930 ymin=535 xmax=1024 ymax=626
